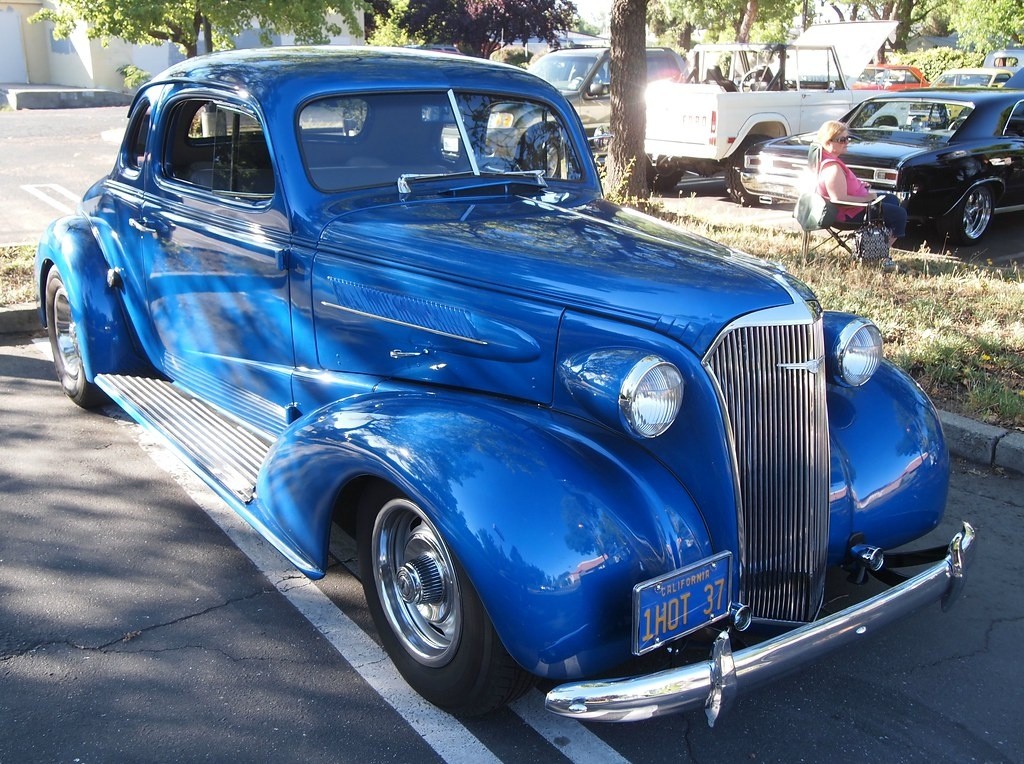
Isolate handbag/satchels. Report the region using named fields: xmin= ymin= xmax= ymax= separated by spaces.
xmin=855 ymin=199 xmax=891 ymax=261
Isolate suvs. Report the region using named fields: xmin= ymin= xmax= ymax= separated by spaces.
xmin=404 ymin=20 xmax=1024 ymax=207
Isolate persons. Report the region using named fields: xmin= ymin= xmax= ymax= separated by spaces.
xmin=814 ymin=120 xmax=906 ymax=273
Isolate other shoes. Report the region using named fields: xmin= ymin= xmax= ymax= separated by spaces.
xmin=880 ymin=257 xmax=895 ymax=269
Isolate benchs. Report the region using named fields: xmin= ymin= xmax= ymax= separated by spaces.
xmin=187 ymin=159 xmax=451 ymax=196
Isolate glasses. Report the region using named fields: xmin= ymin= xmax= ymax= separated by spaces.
xmin=830 ymin=136 xmax=848 ymax=144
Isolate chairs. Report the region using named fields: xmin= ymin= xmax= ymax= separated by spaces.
xmin=701 ymin=64 xmax=738 ymax=92
xmin=791 ymin=162 xmax=886 ymax=268
xmin=749 ymin=66 xmax=780 ymax=91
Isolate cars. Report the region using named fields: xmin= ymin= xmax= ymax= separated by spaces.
xmin=31 ymin=44 xmax=977 ymax=728
xmin=739 ymin=67 xmax=1024 ymax=247
